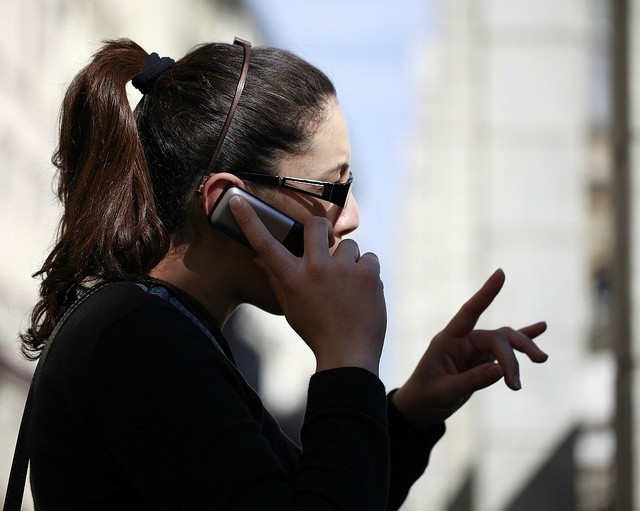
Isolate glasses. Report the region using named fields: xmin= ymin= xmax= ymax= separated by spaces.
xmin=221 ymin=171 xmax=354 ymax=209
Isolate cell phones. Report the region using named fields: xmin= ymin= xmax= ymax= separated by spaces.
xmin=209 ymin=186 xmax=304 ymax=261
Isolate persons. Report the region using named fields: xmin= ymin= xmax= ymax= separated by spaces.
xmin=5 ymin=38 xmax=548 ymax=506
